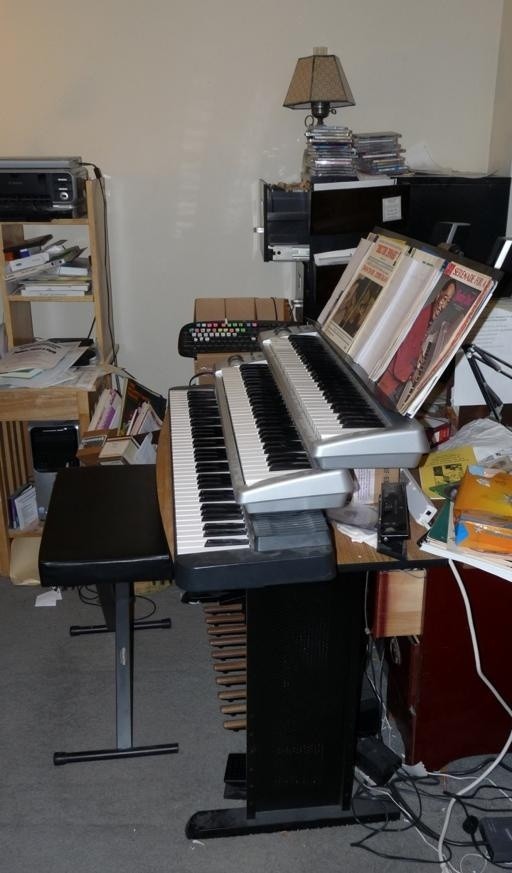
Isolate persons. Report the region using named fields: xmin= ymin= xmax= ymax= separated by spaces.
xmin=376 ymin=278 xmax=460 ymax=395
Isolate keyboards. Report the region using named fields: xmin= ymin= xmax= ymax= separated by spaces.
xmin=178 ymin=320 xmax=304 ymax=360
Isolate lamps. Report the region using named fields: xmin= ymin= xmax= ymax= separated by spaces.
xmin=281 ymin=45 xmax=357 ymax=128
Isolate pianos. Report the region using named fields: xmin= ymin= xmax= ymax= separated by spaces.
xmin=258 ymin=326 xmax=432 ymax=469
xmin=214 ymin=357 xmax=361 ymax=515
xmin=167 ymin=382 xmax=339 ymax=593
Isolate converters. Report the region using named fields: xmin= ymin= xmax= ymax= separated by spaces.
xmin=359 ymin=736 xmax=402 ymax=786
xmin=359 ymin=698 xmax=381 ymax=738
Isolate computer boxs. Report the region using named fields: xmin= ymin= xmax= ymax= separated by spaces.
xmin=28 ymin=420 xmax=82 ymax=522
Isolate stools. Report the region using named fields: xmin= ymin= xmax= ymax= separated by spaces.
xmin=37 ymin=461 xmax=179 ymax=768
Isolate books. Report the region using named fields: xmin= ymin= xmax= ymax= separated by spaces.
xmin=416 ymin=447 xmax=512 ymax=586
xmin=374 ymin=260 xmax=495 ymax=417
xmin=320 ymin=232 xmax=404 ymax=354
xmin=348 ymin=250 xmax=440 ymax=384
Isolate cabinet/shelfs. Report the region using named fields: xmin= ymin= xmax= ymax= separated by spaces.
xmin=0 ymin=174 xmax=120 ymax=577
xmin=256 ymin=168 xmax=511 ymax=323
xmin=366 ymin=404 xmax=512 ymax=771
